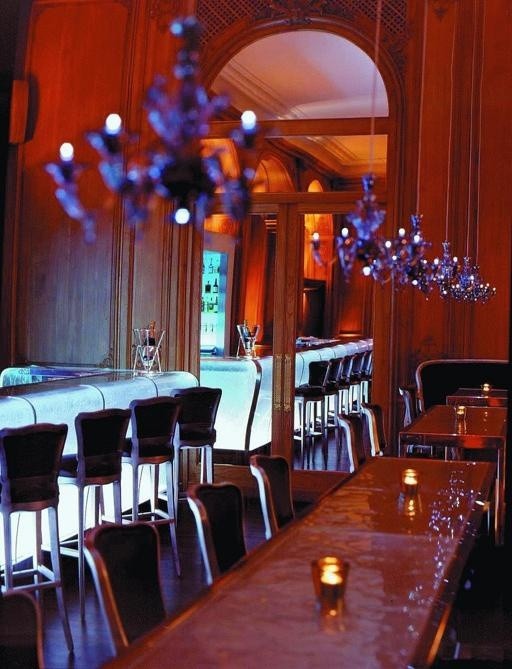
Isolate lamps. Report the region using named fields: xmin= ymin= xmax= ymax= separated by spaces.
xmin=47 ymin=3 xmax=265 ymax=245
xmin=309 ymin=3 xmax=498 ymax=303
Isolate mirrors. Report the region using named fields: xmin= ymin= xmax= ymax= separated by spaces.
xmin=192 ymin=7 xmax=397 ymax=503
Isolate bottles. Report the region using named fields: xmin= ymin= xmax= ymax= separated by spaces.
xmin=142 ymin=320 xmax=156 ymax=361
xmin=241 ymin=318 xmax=251 ymax=343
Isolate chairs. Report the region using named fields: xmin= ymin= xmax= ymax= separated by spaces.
xmin=249 ymin=454 xmax=300 ymax=542
xmin=333 ymin=389 xmax=434 ymax=473
xmin=292 ymin=348 xmax=374 ymax=457
xmin=0 ymin=523 xmax=168 ymax=669
xmin=185 ymin=482 xmax=248 ymax=589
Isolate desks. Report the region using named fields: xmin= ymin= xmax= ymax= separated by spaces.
xmin=398 ymin=388 xmax=511 ymax=549
xmin=103 ymin=454 xmax=501 ymax=668
